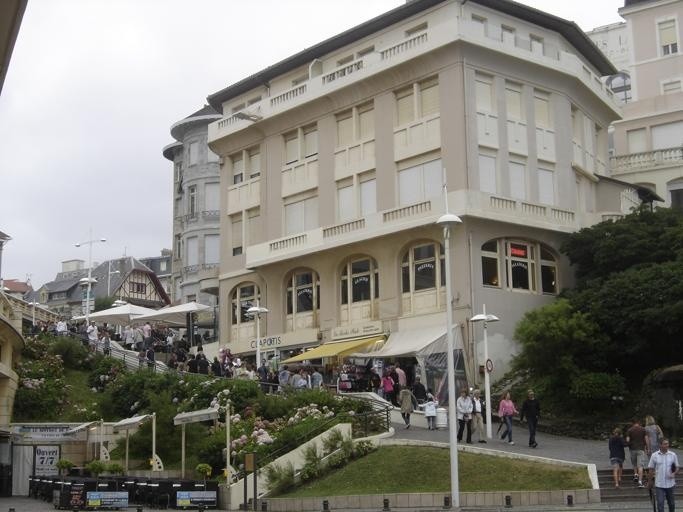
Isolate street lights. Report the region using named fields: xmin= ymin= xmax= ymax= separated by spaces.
xmin=466 ymin=302 xmax=499 ymax=439
xmin=74 ymin=234 xmax=107 ymax=319
xmin=108 ymin=259 xmax=120 ymax=297
xmin=244 ymin=295 xmax=269 ymax=369
xmin=432 ymin=163 xmax=464 ymax=508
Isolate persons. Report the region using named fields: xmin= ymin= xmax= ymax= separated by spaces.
xmin=608 ymin=427 xmax=625 ymax=489
xmin=646 ymin=437 xmax=679 ymax=511
xmin=625 ymin=416 xmax=650 ymax=487
xmin=644 ymin=414 xmax=664 ymax=465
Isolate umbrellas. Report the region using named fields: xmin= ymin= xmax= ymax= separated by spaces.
xmin=643 ymin=480 xmax=656 ymax=511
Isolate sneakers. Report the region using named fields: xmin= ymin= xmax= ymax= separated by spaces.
xmin=458 ymin=437 xmax=538 ymax=448
xmin=615 ymin=477 xmax=646 ymax=491
